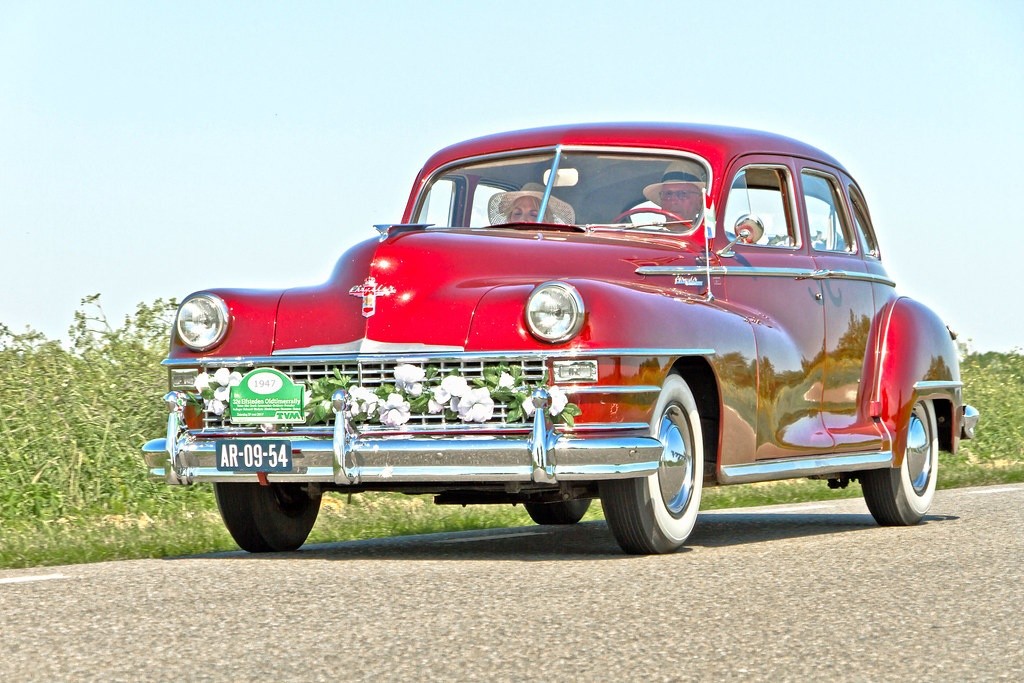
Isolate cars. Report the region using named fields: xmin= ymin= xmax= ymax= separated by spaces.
xmin=139 ymin=120 xmax=982 ymax=555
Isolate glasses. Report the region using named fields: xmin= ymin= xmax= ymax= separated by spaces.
xmin=659 ymin=190 xmax=700 ymax=200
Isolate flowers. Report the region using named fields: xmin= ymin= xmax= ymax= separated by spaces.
xmin=182 ymin=360 xmax=584 ymax=430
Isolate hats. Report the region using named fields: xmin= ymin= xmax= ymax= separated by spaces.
xmin=643 ymin=162 xmax=707 ymax=207
xmin=489 ymin=183 xmax=575 ymax=226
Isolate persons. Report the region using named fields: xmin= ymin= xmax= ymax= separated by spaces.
xmin=642 ymin=160 xmax=706 ymax=231
xmin=488 ymin=182 xmax=575 ymax=225
